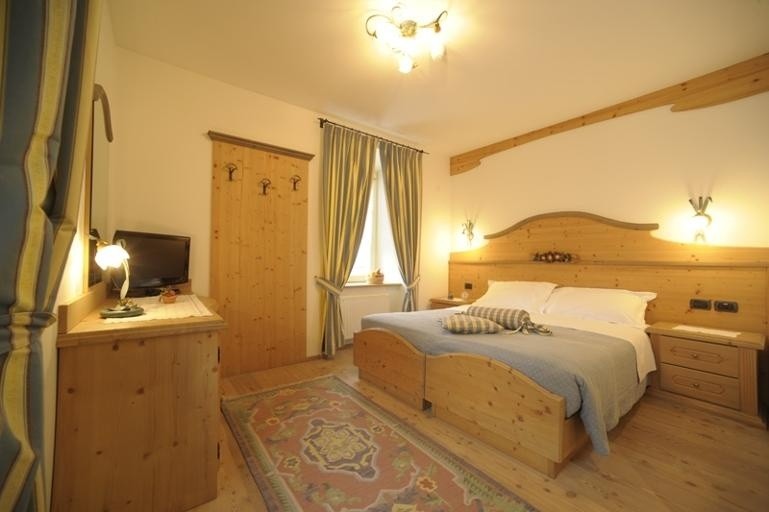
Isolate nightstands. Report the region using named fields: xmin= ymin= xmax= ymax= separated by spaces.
xmin=430 ymin=295 xmax=471 ymax=310
xmin=644 ymin=320 xmax=766 ymax=423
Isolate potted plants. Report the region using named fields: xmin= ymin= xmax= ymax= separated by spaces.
xmin=368 ymin=267 xmax=385 ymax=284
xmin=157 ymin=284 xmax=178 ymax=304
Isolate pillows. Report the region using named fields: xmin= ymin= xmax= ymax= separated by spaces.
xmin=466 ymin=305 xmax=553 ymax=337
xmin=541 ymin=286 xmax=658 ymax=328
xmin=440 ymin=312 xmax=505 ymax=336
xmin=473 ymin=279 xmax=558 ymax=319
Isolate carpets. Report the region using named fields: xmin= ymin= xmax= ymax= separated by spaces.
xmin=222 ymin=373 xmax=542 ymax=511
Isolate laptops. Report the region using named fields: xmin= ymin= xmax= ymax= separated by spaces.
xmin=110 ymin=229 xmax=193 ymax=301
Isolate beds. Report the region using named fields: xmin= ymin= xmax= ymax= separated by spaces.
xmin=352 ymin=305 xmax=652 ymax=482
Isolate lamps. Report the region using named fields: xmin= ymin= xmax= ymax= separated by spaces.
xmin=365 ymin=1 xmax=458 ymax=75
xmin=95 ymin=237 xmax=143 ymax=317
xmin=462 ymin=220 xmax=475 ymax=241
xmin=681 ymin=195 xmax=717 ymax=243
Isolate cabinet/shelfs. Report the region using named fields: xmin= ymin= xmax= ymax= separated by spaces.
xmin=57 ymin=276 xmax=231 ymax=510
